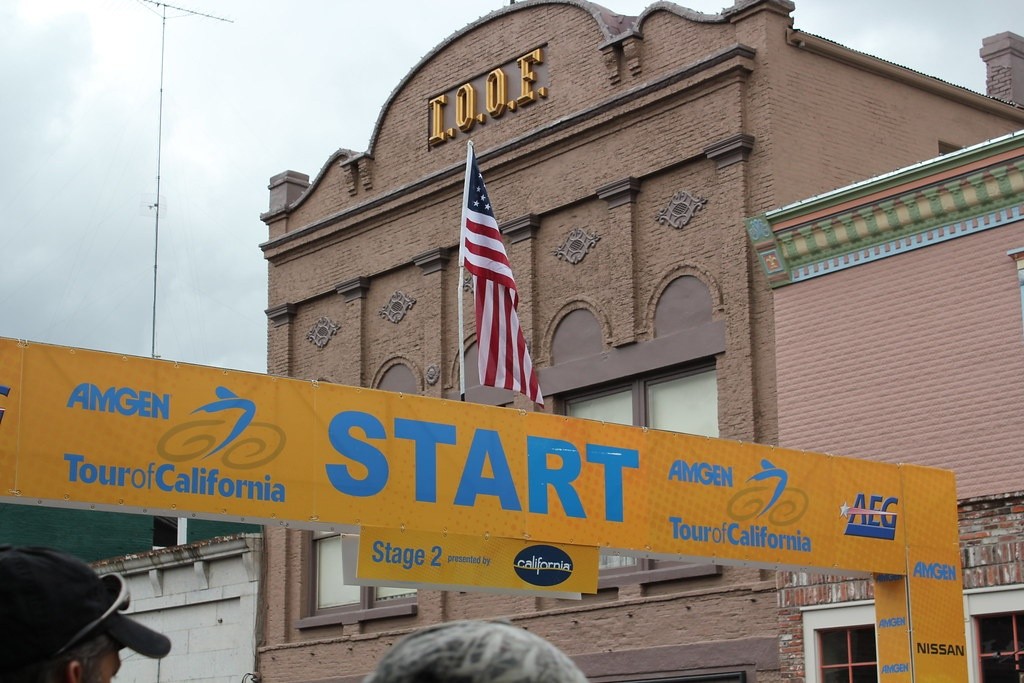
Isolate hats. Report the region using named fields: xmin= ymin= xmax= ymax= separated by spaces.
xmin=0 ymin=543 xmax=172 ymax=659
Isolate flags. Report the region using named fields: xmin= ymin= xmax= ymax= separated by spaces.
xmin=462 ymin=151 xmax=545 ymax=408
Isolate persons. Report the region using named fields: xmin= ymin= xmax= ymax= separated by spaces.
xmin=1 ymin=541 xmax=172 ymax=683
xmin=363 ymin=617 xmax=586 ymax=682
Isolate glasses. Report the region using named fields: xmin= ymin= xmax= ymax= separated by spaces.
xmin=55 ymin=573 xmax=130 ymax=657
